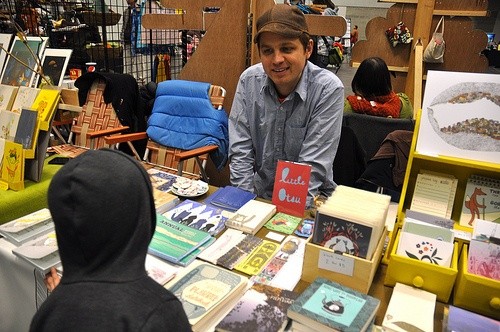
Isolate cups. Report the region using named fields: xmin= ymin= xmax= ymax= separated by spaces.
xmin=86 ymin=62 xmax=97 ymax=72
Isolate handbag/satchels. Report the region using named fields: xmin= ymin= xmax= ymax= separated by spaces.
xmin=422 ymin=16 xmax=445 ymax=64
xmin=385 ymin=22 xmax=412 ymax=48
xmin=328 ymin=47 xmax=343 ymax=65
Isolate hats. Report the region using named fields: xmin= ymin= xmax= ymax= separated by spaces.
xmin=254 ymin=3 xmax=310 ymax=43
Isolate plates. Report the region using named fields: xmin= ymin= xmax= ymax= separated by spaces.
xmin=171 ymin=180 xmax=209 ymax=199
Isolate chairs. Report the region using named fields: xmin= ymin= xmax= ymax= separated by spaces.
xmin=103 ymin=80 xmax=226 ymax=182
xmin=44 ymin=71 xmax=138 ymax=155
xmin=335 ymin=116 xmax=416 ymax=208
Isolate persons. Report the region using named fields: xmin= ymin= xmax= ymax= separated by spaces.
xmin=227 ymin=4 xmax=345 ymax=206
xmin=351 ymin=25 xmax=358 ymax=42
xmin=29 ymin=149 xmax=193 ymax=332
xmin=343 ymin=57 xmax=413 ymax=119
xmin=121 ymin=0 xmax=139 ymax=75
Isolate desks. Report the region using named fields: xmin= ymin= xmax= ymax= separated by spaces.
xmin=1 ymin=141 xmax=500 ymax=332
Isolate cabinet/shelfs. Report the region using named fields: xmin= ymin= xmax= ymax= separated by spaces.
xmin=385 ymin=110 xmax=500 ymax=322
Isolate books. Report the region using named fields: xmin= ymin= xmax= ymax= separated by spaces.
xmin=286 ymin=171 xmax=500 ymax=332
xmin=145 ymin=186 xmax=308 ymax=332
xmin=0 ymin=208 xmax=61 ymax=274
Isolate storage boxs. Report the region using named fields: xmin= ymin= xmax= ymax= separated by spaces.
xmin=301 ymin=226 xmax=386 ymax=295
